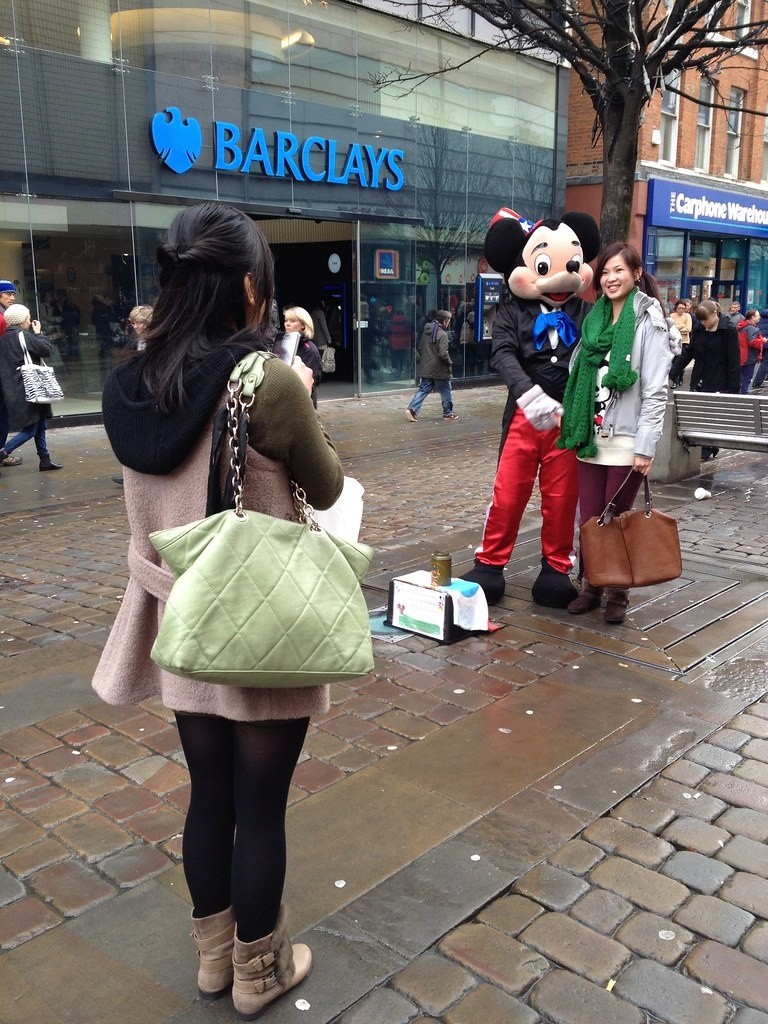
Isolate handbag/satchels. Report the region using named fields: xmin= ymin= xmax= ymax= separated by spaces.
xmin=320 ymin=347 xmax=336 ymax=372
xmin=580 ymin=465 xmax=682 ymax=588
xmin=16 ymin=331 xmax=64 ymax=404
xmin=147 ymin=349 xmax=374 ymax=687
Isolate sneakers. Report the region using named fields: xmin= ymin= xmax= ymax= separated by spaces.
xmin=0 ymin=455 xmax=23 ymax=467
xmin=405 ymin=410 xmax=418 ymax=421
xmin=443 ymin=414 xmax=458 ymax=420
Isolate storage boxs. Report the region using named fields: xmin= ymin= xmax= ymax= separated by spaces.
xmin=384 ymin=577 xmax=481 ymax=645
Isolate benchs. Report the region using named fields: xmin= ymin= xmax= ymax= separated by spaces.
xmin=672 ymin=390 xmax=768 ymax=454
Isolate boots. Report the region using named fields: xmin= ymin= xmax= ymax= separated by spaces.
xmin=604 ymin=587 xmax=630 ymax=622
xmin=567 ymin=577 xmax=604 ymax=613
xmin=39 ymin=457 xmax=64 ymax=471
xmin=191 ymin=903 xmax=234 ymax=1001
xmin=232 ymin=902 xmax=313 ymax=1020
xmin=0 ymin=448 xmax=9 ymax=463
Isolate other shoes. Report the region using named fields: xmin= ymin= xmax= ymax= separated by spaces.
xmin=679 ymin=379 xmax=683 ymax=386
xmin=752 ymin=382 xmax=764 ymax=388
xmin=671 ymin=383 xmax=677 ymax=388
xmin=113 ymin=475 xmax=124 ymax=484
xmin=765 ymin=378 xmax=768 ymax=380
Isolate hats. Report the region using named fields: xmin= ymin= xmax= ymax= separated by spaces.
xmin=4 ymin=304 xmax=29 ymax=325
xmin=0 ymin=280 xmax=16 ymax=295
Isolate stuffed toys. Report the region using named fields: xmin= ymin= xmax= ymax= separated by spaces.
xmin=458 ymin=207 xmax=682 ymax=610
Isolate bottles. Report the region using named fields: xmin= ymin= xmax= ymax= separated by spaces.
xmin=432 ymin=551 xmax=451 ymax=586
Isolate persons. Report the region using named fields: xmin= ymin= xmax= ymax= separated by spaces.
xmin=0 ymin=304 xmax=64 ymax=471
xmin=670 ymin=300 xmax=692 ymax=389
xmin=283 ymin=306 xmax=322 ymax=409
xmin=724 ymin=300 xmax=745 ymax=326
xmin=454 ymin=301 xmax=476 ymax=375
xmin=90 ymin=295 xmax=120 ymax=380
xmin=405 ymin=310 xmax=458 ymax=421
xmin=685 ymin=298 xmax=697 ymax=328
xmin=93 ymin=202 xmax=344 ymax=1021
xmin=737 ymin=309 xmax=768 ymax=394
xmin=360 ymin=294 xmax=437 ymax=386
xmin=129 ymin=305 xmax=154 ymax=351
xmin=0 ymin=280 xmax=23 ymax=466
xmin=669 ymin=300 xmax=741 ymax=461
xmin=752 ymin=309 xmax=768 ymax=388
xmin=310 ymin=298 xmax=331 ymax=383
xmin=556 ymin=242 xmax=674 ymax=623
xmin=39 ymin=288 xmax=82 ymax=378
